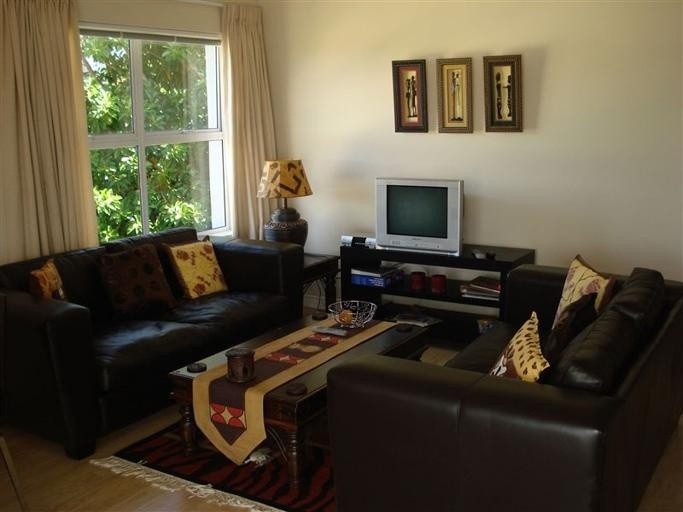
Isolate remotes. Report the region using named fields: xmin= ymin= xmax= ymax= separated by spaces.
xmin=472 ymin=249 xmax=487 ymax=260
xmin=485 ymin=252 xmax=497 ymax=259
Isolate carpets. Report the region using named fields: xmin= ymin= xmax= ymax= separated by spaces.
xmin=89 ymin=415 xmax=338 ymax=512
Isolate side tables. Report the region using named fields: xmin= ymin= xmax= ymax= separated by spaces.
xmin=303 ymin=253 xmax=340 ymax=317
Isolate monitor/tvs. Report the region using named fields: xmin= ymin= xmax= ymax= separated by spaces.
xmin=376 ymin=178 xmax=465 ymax=258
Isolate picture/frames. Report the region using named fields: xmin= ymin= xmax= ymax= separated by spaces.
xmin=392 ymin=59 xmax=428 ymax=133
xmin=436 ymin=57 xmax=473 ymax=133
xmin=483 ymin=55 xmax=523 ymax=131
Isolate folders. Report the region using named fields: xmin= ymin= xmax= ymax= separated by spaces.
xmin=460 ymin=276 xmax=500 ymax=301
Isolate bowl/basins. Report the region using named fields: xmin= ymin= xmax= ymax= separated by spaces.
xmin=327 ymin=299 xmax=378 ymax=328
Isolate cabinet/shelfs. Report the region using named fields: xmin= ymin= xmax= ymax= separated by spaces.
xmin=340 ymin=244 xmax=536 ymax=352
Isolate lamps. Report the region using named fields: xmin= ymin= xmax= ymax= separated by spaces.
xmin=257 ymin=160 xmax=313 ymax=253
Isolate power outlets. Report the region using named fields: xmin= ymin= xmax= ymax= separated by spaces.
xmin=336 ymin=266 xmax=341 ymax=278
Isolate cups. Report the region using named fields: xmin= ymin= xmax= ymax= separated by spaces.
xmin=224 ymin=347 xmax=259 ymax=385
xmin=409 ymin=271 xmax=425 ymax=290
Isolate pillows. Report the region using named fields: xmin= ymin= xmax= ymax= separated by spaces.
xmin=550 ymin=254 xmax=614 ymax=353
xmin=167 ymin=235 xmax=228 ymax=300
xmin=106 ymin=244 xmax=168 ymax=315
xmin=31 ymin=259 xmax=67 ymax=300
xmin=489 ymin=311 xmax=550 ymax=383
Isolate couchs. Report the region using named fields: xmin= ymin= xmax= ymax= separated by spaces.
xmin=0 ymin=227 xmax=305 ymax=460
xmin=327 ymin=265 xmax=683 ymax=512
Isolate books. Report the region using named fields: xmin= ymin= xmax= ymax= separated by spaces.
xmin=459 ymin=276 xmax=500 ymax=301
xmin=350 ymin=265 xmax=398 ymax=277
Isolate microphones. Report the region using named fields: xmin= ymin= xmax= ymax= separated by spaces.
xmin=311 ymin=326 xmax=347 ymax=336
